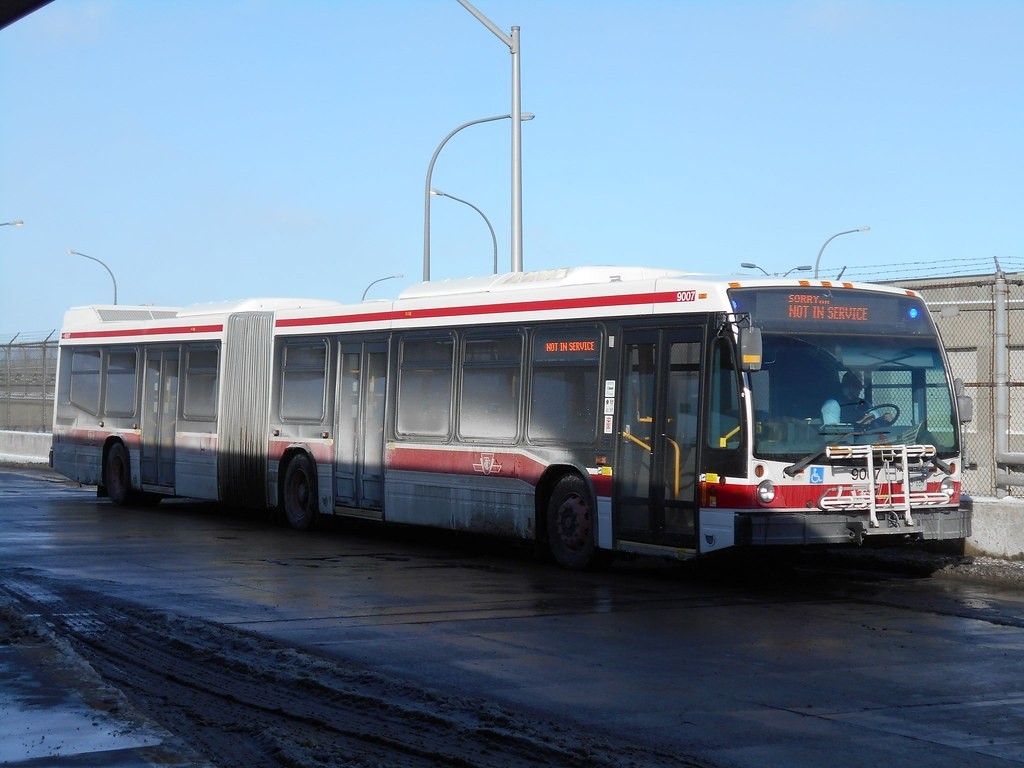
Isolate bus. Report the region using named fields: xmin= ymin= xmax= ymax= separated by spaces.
xmin=49 ymin=264 xmax=973 ymax=584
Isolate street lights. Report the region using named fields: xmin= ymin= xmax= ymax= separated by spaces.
xmin=362 ymin=274 xmax=404 ymax=301
xmin=65 ymin=249 xmax=117 ymax=305
xmin=428 ymin=185 xmax=498 ymax=275
xmin=421 ymin=112 xmax=535 ymax=282
xmin=815 ymin=226 xmax=870 ymax=279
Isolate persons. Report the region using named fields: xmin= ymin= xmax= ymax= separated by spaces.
xmin=821 ymin=369 xmax=894 ymax=427
